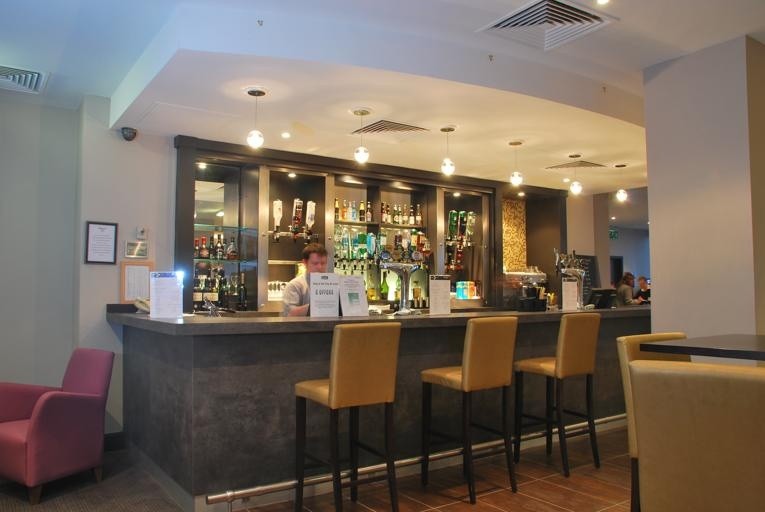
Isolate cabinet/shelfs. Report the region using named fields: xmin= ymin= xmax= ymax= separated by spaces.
xmin=193 ymin=221 xmax=246 ymax=265
xmin=335 ymin=220 xmax=431 ymax=267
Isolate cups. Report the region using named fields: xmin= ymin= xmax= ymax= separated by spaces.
xmin=504 ymin=265 xmax=541 ymax=273
xmin=268 ymin=282 xmax=287 ymax=298
xmin=413 ymin=298 xmax=423 ymax=308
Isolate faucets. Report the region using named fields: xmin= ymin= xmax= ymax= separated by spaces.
xmin=202 ymin=296 xmax=239 ymax=318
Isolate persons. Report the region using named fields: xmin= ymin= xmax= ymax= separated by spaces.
xmin=611 ymin=272 xmax=650 ymax=307
xmin=280 ymin=242 xmax=328 ymax=317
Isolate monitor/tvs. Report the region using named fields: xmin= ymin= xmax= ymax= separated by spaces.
xmin=584 ymin=288 xmax=617 ymax=308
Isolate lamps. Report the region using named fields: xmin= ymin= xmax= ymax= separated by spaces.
xmin=615 ymin=164 xmax=629 ymax=203
xmin=246 ymin=89 xmax=265 ymax=150
xmin=354 ymin=109 xmax=370 ymax=164
xmin=508 ymin=142 xmax=523 ymax=187
xmin=440 ymin=127 xmax=456 ymax=176
xmin=568 ymin=154 xmax=587 ymax=196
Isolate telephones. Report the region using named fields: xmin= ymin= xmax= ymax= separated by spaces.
xmin=133 ymin=298 xmax=150 ymax=313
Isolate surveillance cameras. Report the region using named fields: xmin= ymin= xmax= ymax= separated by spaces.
xmin=122 ymin=127 xmax=138 ymax=140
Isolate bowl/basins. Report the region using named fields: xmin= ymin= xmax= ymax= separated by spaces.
xmin=520 ymin=299 xmax=547 ymax=311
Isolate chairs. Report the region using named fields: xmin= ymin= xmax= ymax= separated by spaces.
xmin=292 ymin=322 xmax=401 ymax=511
xmin=420 ymin=317 xmax=519 ymax=505
xmin=0 ymin=345 xmax=118 ymax=505
xmin=616 ymin=332 xmax=694 ymax=512
xmin=628 ymin=359 xmax=764 ymax=512
xmin=513 ymin=313 xmax=603 ymax=480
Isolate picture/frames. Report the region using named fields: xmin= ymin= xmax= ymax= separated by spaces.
xmin=120 ymin=260 xmax=156 ymax=306
xmin=85 ymin=220 xmax=119 ymax=265
xmin=124 ymin=239 xmax=149 ymax=259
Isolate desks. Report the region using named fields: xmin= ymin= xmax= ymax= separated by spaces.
xmin=639 ymin=333 xmax=764 ymax=361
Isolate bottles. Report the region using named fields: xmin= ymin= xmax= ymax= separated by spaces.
xmin=272 ymin=200 xmax=282 ymax=238
xmin=293 ymin=199 xmax=303 ymax=237
xmin=193 ymin=234 xmax=247 ymax=309
xmin=446 ymin=210 xmax=476 ymax=272
xmin=368 ymin=274 xmax=376 ymax=301
xmin=381 ymin=272 xmax=389 ymax=301
xmin=396 ymin=274 xmax=402 ymax=301
xmin=335 ymin=195 xmax=434 ymax=272
xmin=456 ymin=281 xmax=476 ymax=297
xmin=306 ymin=201 xmax=317 ymax=240
xmin=412 ymin=280 xmax=421 ymax=298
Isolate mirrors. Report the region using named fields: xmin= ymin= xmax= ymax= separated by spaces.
xmin=194 ymin=179 xmax=238 ymax=280
